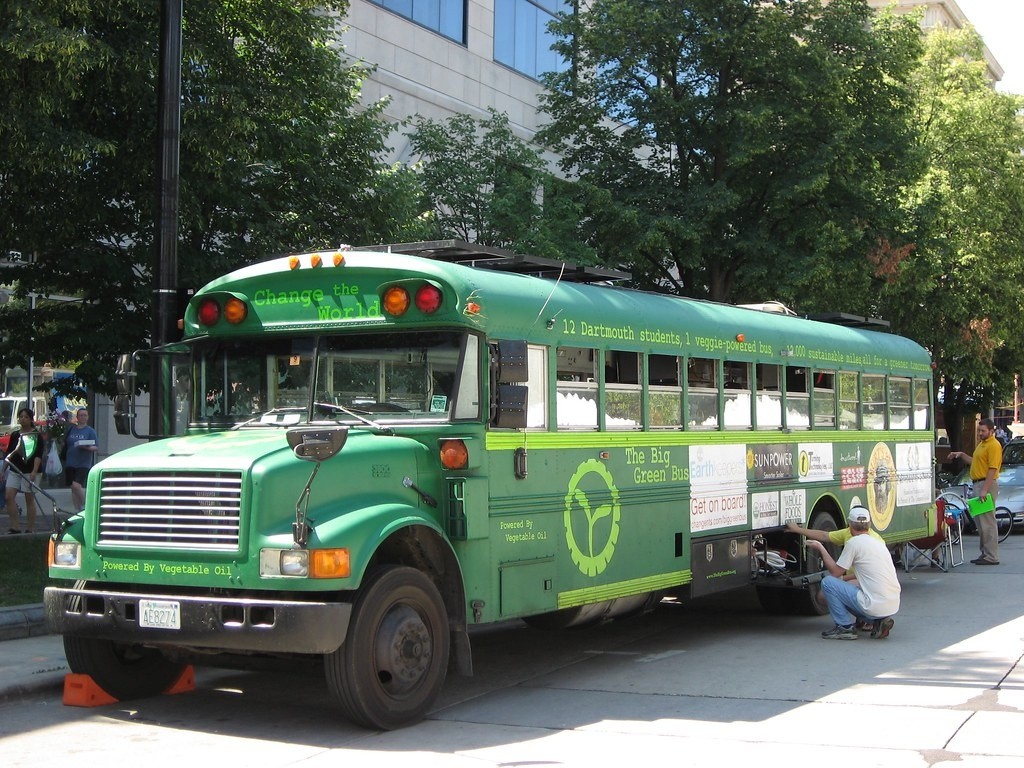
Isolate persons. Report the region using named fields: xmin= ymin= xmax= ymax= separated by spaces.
xmin=994 ymin=425 xmax=1004 ymax=447
xmin=783 ymin=505 xmax=902 ymax=640
xmin=60 ymin=408 xmax=99 ymax=513
xmin=0 ymin=407 xmax=44 ymax=535
xmin=946 ymin=417 xmax=1003 ymax=566
xmin=931 ymin=488 xmax=945 ymax=568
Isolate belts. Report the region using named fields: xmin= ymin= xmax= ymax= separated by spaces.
xmin=973 ymin=478 xmax=985 ymax=483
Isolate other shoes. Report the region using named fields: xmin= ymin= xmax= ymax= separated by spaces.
xmin=862 ymin=623 xmax=873 ymax=630
xmin=971 ymin=559 xmax=981 ymax=563
xmin=976 ymin=559 xmax=999 ymax=565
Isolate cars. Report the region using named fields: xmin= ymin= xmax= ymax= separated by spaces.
xmin=1002 ymin=436 xmax=1024 ymax=466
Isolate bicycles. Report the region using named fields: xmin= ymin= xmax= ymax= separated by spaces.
xmin=935 ymin=477 xmax=1013 ymax=545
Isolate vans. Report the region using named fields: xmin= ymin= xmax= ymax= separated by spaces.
xmin=0 ymin=397 xmax=48 ymax=454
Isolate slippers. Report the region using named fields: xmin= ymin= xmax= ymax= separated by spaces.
xmin=8 ymin=528 xmax=21 ymax=534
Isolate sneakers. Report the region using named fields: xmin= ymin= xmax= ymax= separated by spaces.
xmin=871 ymin=617 xmax=894 ymax=639
xmin=822 ymin=625 xmax=858 ymax=640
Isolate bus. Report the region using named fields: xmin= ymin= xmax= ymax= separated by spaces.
xmin=2 ymin=238 xmax=940 ymax=731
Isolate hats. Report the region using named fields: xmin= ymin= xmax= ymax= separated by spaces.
xmin=847 ymin=508 xmax=870 ymax=522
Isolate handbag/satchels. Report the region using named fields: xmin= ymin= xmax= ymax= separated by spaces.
xmin=45 ymin=440 xmax=63 ymax=475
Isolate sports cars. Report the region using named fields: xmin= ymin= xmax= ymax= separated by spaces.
xmin=936 ymin=463 xmax=1024 ymax=529
